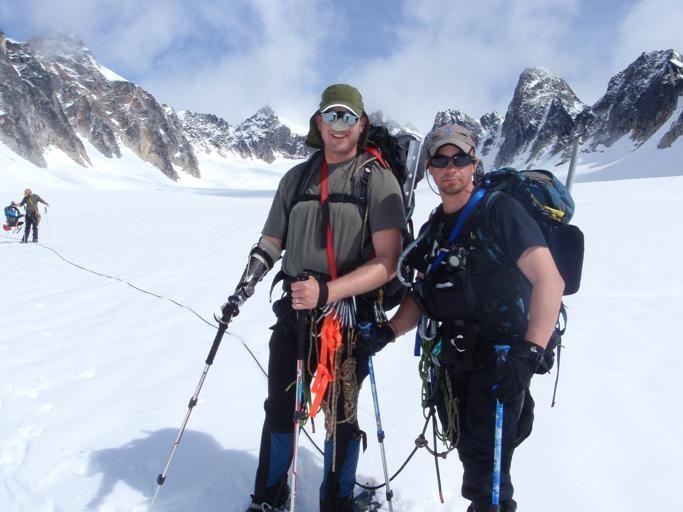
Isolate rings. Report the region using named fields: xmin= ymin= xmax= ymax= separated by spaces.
xmin=295 ymin=298 xmax=298 ymax=304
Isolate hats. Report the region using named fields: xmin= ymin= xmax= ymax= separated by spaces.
xmin=319 ymin=84 xmax=364 ymax=119
xmin=428 ymin=123 xmax=475 ymax=156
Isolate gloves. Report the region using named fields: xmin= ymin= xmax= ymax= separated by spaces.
xmin=359 ymin=320 xmax=396 ymax=354
xmin=488 ymin=338 xmax=546 ymax=404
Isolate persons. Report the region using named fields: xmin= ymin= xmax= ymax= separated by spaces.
xmin=220 ymin=82 xmax=407 ymax=509
xmin=358 ymin=122 xmax=568 ymax=511
xmin=11 ymin=189 xmax=51 ymax=243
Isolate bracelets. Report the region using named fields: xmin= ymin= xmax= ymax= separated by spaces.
xmin=315 ymin=276 xmax=330 ymax=309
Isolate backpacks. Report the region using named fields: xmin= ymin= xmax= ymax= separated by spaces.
xmin=477 ymin=167 xmax=587 ymax=378
xmin=291 ymin=122 xmax=427 ymax=312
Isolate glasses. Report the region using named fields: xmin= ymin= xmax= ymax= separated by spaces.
xmin=429 ymin=154 xmax=475 ymax=168
xmin=319 ymin=109 xmax=357 ymax=126
